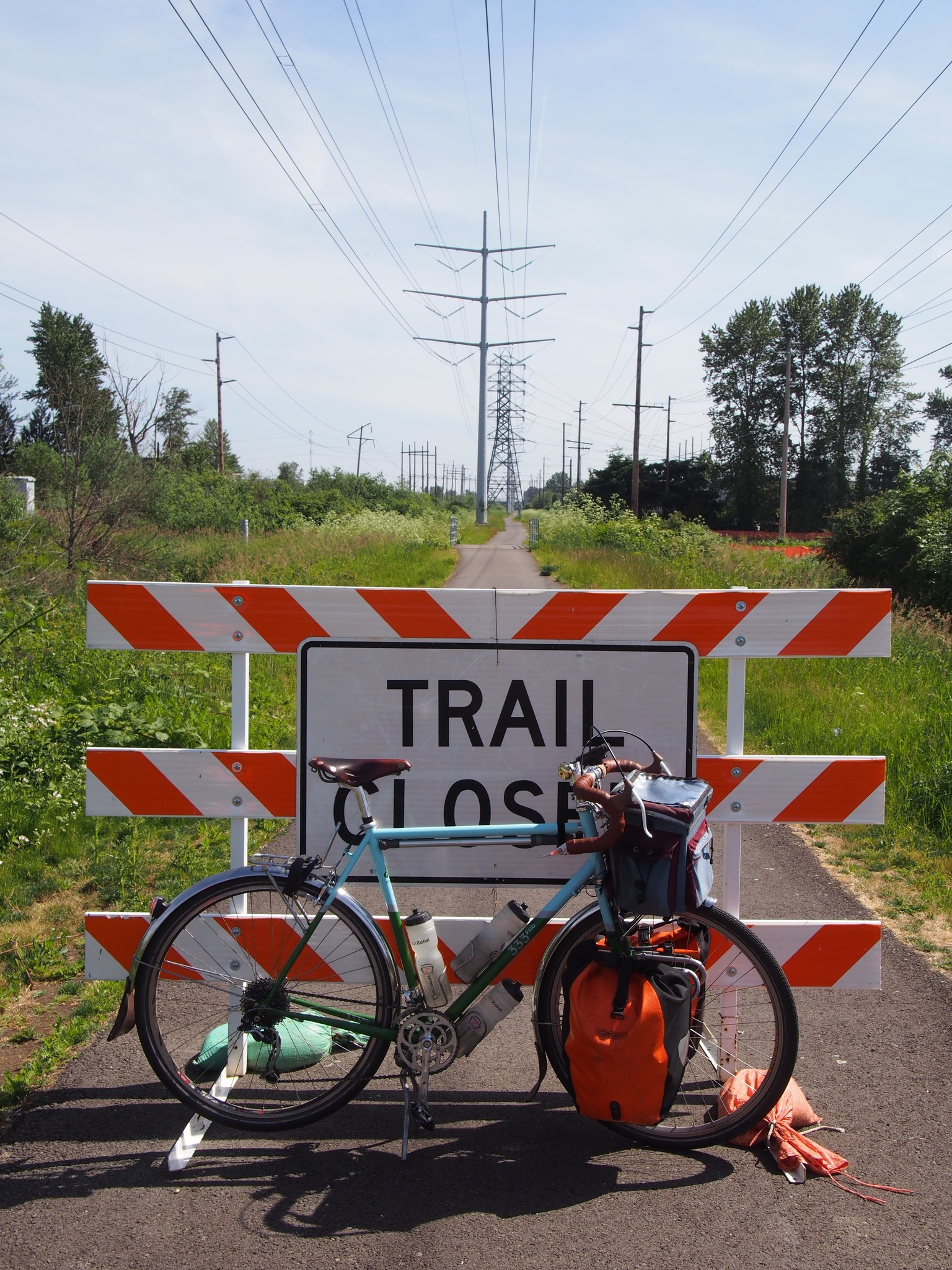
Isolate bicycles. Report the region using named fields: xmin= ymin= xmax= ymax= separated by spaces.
xmin=108 ymin=727 xmax=799 ymax=1176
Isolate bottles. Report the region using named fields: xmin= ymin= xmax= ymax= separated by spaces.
xmin=450 ymin=900 xmax=530 ymax=983
xmin=405 ymin=908 xmax=452 ymax=1007
xmin=442 ymin=979 xmax=524 ymax=1058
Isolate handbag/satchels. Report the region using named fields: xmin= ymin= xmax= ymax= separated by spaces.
xmin=562 ymin=927 xmax=706 ymax=1121
xmin=608 ymin=769 xmax=715 ymax=916
